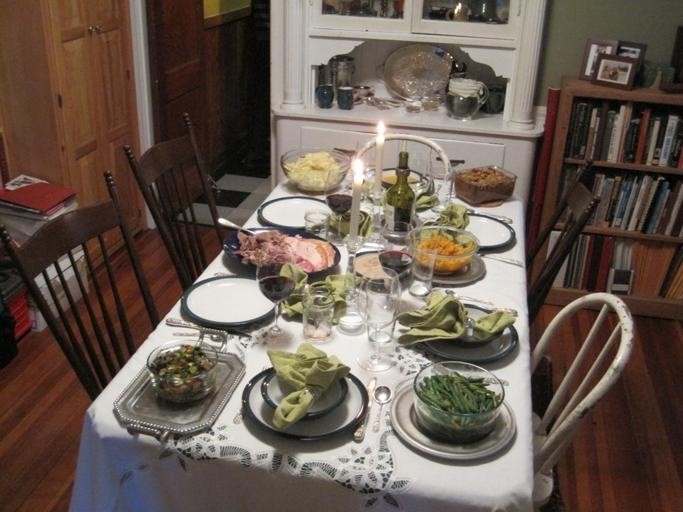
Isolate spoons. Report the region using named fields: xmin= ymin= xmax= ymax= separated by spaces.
xmin=370 ymin=384 xmax=392 ymax=434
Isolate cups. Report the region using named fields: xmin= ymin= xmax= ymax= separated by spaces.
xmin=314 ymin=83 xmax=334 ymax=109
xmin=335 ymin=288 xmax=365 ymax=335
xmin=300 ymin=289 xmax=334 ymax=344
xmin=410 ymin=236 xmax=439 ymax=298
xmin=336 ymin=86 xmax=353 ymax=110
xmin=486 ymin=83 xmax=504 ymax=114
xmin=304 ymin=210 xmax=330 ymax=240
xmin=428 ymin=166 xmax=454 ymax=213
xmin=355 ymin=85 xmax=369 ymax=97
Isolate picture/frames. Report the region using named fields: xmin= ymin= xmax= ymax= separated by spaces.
xmin=581 ymin=38 xmax=614 ymax=80
xmin=590 ymin=53 xmax=639 ymax=91
xmin=616 ymin=39 xmax=646 ymax=72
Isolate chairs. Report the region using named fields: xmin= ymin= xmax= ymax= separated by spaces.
xmin=5 ymin=170 xmax=161 ymax=400
xmin=523 ymin=156 xmax=603 ymax=319
xmin=530 ymin=291 xmax=635 ymax=505
xmin=121 ymin=113 xmax=232 ymax=292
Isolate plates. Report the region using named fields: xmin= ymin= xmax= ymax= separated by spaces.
xmin=461 ymin=214 xmax=515 ymax=252
xmin=179 ymin=274 xmax=273 ymax=329
xmin=382 ymin=41 xmax=463 ymax=105
xmin=256 ymin=195 xmax=331 ymax=234
xmin=408 ymin=257 xmax=485 ymax=287
xmin=239 ymin=363 xmax=368 ymax=442
xmin=421 ymin=304 xmax=518 ymax=363
xmin=353 ymin=93 xmax=375 ymax=101
xmin=388 ymin=381 xmax=516 ymax=462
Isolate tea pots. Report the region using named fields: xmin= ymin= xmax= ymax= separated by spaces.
xmin=444 ymin=77 xmax=489 ymax=121
xmin=326 ymin=52 xmax=354 ymax=97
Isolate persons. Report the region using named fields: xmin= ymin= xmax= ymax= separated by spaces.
xmin=609 ymin=67 xmax=618 ymax=81
xmin=601 ymin=66 xmax=610 ymax=79
xmin=590 ymin=46 xmax=607 ymax=74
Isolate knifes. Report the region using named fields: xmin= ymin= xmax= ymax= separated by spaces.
xmin=161 ymin=316 xmax=252 ymax=340
xmin=351 ymin=377 xmax=376 ymax=444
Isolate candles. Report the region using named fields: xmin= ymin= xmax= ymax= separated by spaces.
xmin=346 ymin=158 xmax=364 ymax=236
xmin=373 ymin=120 xmax=385 ymax=193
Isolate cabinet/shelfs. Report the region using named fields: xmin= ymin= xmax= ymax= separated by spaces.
xmin=270 ymin=104 xmax=543 ymax=199
xmin=535 ymin=74 xmax=682 ymax=320
xmin=0 ymin=1 xmax=148 ymax=282
xmin=308 ymin=0 xmax=527 ymax=40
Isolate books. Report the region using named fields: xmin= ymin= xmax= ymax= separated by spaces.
xmin=0 ymin=174 xmax=90 ymax=334
xmin=544 ymin=94 xmax=681 ymax=304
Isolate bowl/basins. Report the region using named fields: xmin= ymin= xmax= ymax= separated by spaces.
xmin=408 ymin=226 xmax=480 ymax=277
xmin=379 ymin=167 xmax=423 ymax=187
xmin=412 ymin=360 xmax=505 ymax=443
xmin=260 ymin=367 xmax=349 ymax=419
xmin=147 ymin=338 xmax=219 ymax=402
xmin=279 ymin=146 xmax=352 ymax=193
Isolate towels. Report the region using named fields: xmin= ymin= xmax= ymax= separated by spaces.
xmin=391 ymin=189 xmax=447 ymax=208
xmin=278 ymin=262 xmax=358 ymax=324
xmin=326 ymin=208 xmax=372 ymax=233
xmin=422 ymin=205 xmax=474 ymax=235
xmin=264 ymin=344 xmax=355 ymax=428
xmin=401 ymin=294 xmax=518 ymax=349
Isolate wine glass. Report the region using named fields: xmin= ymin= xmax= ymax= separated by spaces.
xmin=323 ymin=167 xmax=353 ymax=246
xmin=253 ymin=247 xmax=296 ymax=345
xmin=354 ymin=266 xmax=402 ymax=373
xmin=378 ymin=221 xmax=415 ymax=307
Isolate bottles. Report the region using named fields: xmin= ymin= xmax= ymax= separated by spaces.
xmin=380 ymin=150 xmax=415 ymax=236
